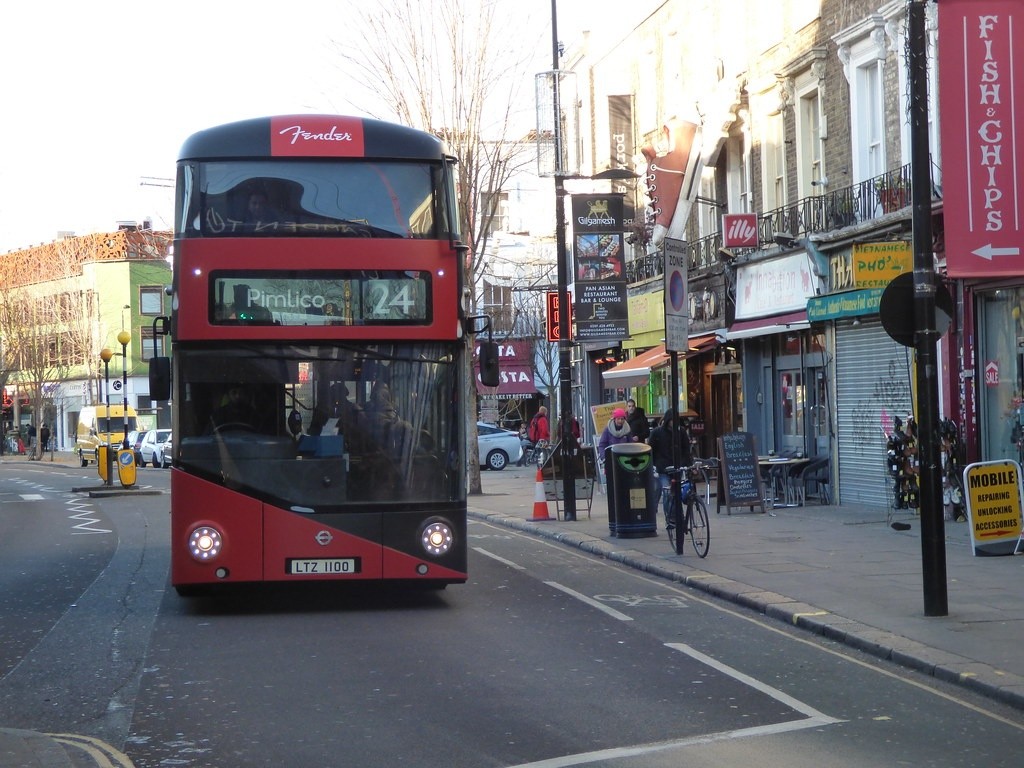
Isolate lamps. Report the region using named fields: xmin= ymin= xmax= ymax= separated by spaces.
xmin=717 ymin=247 xmax=736 ymax=264
xmin=773 ymin=232 xmax=799 ymax=248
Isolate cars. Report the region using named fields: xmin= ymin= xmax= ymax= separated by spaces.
xmin=477 ymin=421 xmax=525 ymax=471
xmin=118 ymin=429 xmax=175 ymax=469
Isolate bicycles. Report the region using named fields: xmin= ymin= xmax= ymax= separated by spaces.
xmin=522 ymin=439 xmax=553 ymax=467
xmin=665 ymin=464 xmax=711 ymax=558
xmin=28 ymin=440 xmax=46 ymax=462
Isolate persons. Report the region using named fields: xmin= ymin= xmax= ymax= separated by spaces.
xmin=235 ymin=190 xmax=287 ymax=229
xmin=519 ymin=421 xmax=529 ymax=441
xmin=26 ymin=424 xmax=35 ymax=445
xmin=625 ymin=399 xmax=650 ymax=443
xmin=648 ymin=409 xmax=698 ymax=528
xmin=41 ymin=424 xmax=49 ymax=452
xmin=203 ymin=385 xmax=260 ymax=433
xmin=598 ymin=409 xmax=633 ymax=462
xmin=529 ymin=406 xmax=550 ymax=442
xmin=557 ymin=410 xmax=580 ymax=441
xmin=332 ymin=382 xmax=431 ymax=457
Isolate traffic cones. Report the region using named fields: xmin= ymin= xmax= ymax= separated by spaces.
xmin=523 ymin=467 xmax=556 ymax=521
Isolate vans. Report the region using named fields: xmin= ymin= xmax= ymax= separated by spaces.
xmin=75 ymin=405 xmax=145 ymax=467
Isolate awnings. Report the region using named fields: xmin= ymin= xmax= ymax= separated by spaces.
xmin=602 ymin=337 xmax=716 ymax=389
xmin=474 ymin=365 xmax=538 ymax=399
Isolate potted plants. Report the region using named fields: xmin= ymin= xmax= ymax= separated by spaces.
xmin=829 ymin=194 xmax=856 ymax=228
xmin=875 ymin=176 xmax=912 ymax=215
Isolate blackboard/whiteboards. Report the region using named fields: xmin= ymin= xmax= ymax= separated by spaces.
xmin=715 ymin=433 xmax=763 ymax=505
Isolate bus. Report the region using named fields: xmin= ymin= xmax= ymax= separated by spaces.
xmin=149 ymin=115 xmax=500 ymax=596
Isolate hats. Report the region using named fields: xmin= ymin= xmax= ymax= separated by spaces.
xmin=612 ymin=409 xmax=626 ymax=419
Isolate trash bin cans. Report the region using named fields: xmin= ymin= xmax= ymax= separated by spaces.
xmin=603 ymin=443 xmax=657 ymax=538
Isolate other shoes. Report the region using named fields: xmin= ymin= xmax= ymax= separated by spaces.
xmin=664 ymin=521 xmax=675 ymax=529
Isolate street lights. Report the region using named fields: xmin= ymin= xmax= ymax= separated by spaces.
xmin=550 ymin=1 xmax=641 ymax=519
xmin=100 ymin=330 xmax=131 ymax=489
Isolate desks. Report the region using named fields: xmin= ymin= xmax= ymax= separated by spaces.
xmin=759 ymin=458 xmax=809 ymax=507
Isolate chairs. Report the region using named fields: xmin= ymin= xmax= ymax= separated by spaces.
xmin=693 ymin=451 xmax=830 ymax=508
